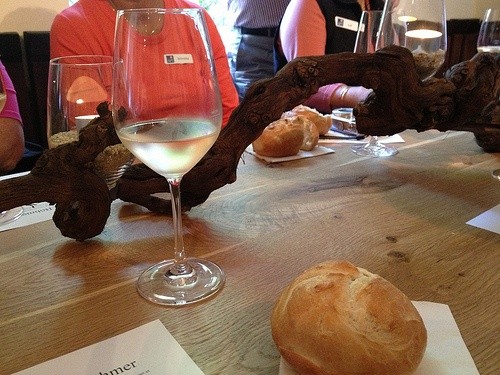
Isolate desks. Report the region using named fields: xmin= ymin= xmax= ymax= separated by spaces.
xmin=0 ymin=130 xmax=500 ymax=375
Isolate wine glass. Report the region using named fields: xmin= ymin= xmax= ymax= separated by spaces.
xmin=0 ymin=70 xmax=24 ymax=227
xmin=110 ymin=8 xmax=228 ymax=308
xmin=350 ymin=11 xmax=406 ymax=158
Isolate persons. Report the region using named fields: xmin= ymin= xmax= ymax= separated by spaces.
xmin=224 ymin=0 xmax=290 ymax=98
xmin=0 ymin=60 xmax=25 ymax=170
xmin=50 ymin=0 xmax=240 ymax=130
xmin=279 ymin=0 xmax=399 ymax=114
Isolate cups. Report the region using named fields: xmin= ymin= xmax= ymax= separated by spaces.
xmin=48 ymin=54 xmax=136 ymax=190
xmin=477 ymin=8 xmax=500 ymax=53
xmin=375 ymin=0 xmax=447 ymax=82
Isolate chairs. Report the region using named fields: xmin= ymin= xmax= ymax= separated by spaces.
xmin=0 ymin=31 xmax=50 ymax=177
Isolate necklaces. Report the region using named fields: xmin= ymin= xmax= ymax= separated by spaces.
xmin=116 ymin=8 xmax=161 ymax=47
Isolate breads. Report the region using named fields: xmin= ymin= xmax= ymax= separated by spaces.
xmin=253 ymin=105 xmax=331 ymax=157
xmin=272 ymin=259 xmax=427 ymax=375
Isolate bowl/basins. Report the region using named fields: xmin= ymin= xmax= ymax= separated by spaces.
xmin=331 ymin=108 xmax=361 ymax=136
